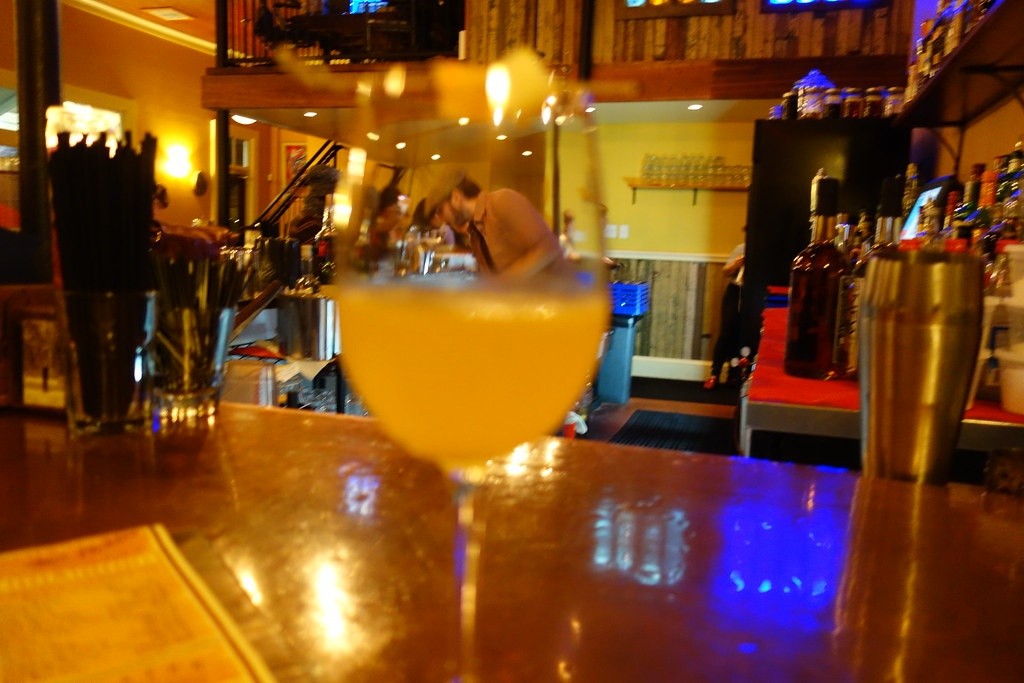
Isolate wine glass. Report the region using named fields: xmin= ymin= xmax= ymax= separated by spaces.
xmin=333 ymin=96 xmax=605 ymax=683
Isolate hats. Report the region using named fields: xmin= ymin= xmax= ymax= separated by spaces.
xmin=421 ymin=171 xmax=463 ymax=217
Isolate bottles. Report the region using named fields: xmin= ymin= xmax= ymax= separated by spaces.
xmin=293 ymin=244 xmax=321 ymax=294
xmin=770 ymin=1 xmax=1024 ymax=374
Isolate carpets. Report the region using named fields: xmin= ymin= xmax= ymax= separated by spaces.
xmin=607 ymin=409 xmax=738 ymax=456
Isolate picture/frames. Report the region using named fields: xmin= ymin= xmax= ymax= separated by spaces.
xmin=282 ymin=143 xmax=308 ymax=198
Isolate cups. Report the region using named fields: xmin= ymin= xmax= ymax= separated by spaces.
xmin=217 ymin=359 xmax=278 ymax=408
xmin=639 ymin=150 xmax=751 ymax=184
xmin=859 ymin=248 xmax=987 ymax=490
xmin=147 ymin=292 xmax=236 ymax=427
xmin=52 ymin=289 xmax=147 ymax=435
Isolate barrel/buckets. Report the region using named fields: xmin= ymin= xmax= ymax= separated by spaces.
xmin=276 ymin=294 xmax=341 ymax=361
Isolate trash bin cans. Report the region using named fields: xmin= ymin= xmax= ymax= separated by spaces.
xmin=597 ymin=315 xmax=643 ymax=404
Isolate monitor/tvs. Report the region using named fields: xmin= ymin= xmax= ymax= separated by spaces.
xmin=901 ymin=176 xmax=956 ymax=241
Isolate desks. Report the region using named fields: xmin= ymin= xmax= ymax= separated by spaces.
xmin=742 ymin=286 xmax=1024 ymax=451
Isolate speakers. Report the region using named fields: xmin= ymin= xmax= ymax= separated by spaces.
xmin=811 ymin=176 xmax=838 ymax=217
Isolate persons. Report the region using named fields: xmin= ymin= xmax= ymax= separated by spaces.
xmin=435 ymin=224 xmax=454 ymax=252
xmin=243 ymin=219 xmax=280 ymax=299
xmin=704 ymin=223 xmax=746 ymax=389
xmin=400 ymin=198 xmax=478 ymax=274
xmin=366 ymin=184 xmax=403 ymax=246
xmin=423 ymin=169 xmax=565 ymax=285
